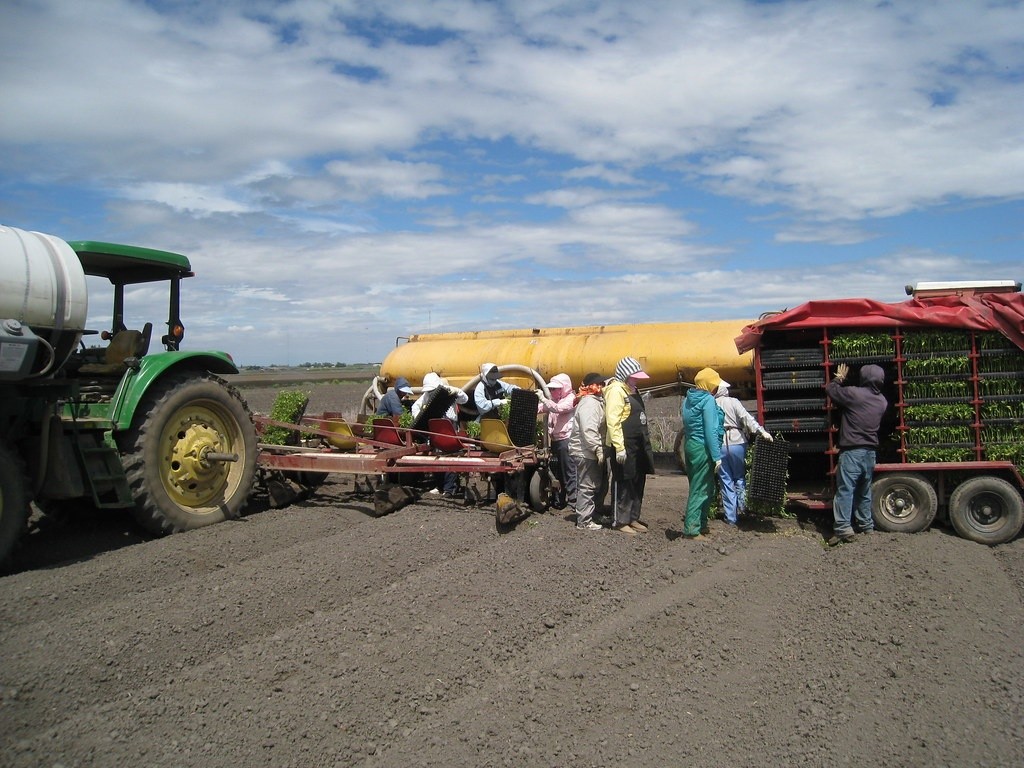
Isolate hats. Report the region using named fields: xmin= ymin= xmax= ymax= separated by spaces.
xmin=547 ymin=382 xmax=563 ymax=388
xmin=584 ymin=373 xmax=604 ymax=385
xmin=399 ymin=386 xmax=414 ymax=396
xmin=718 ymin=380 xmax=731 ymax=387
xmin=630 ymin=371 xmax=650 ymax=379
xmin=487 ymin=367 xmax=503 ymax=379
xmin=422 ymin=372 xmax=440 ymax=391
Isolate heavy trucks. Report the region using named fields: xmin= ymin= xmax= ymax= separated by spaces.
xmin=734 ymin=280 xmax=1024 ymax=547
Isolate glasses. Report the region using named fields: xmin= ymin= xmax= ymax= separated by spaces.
xmin=600 ymin=382 xmax=605 ymax=386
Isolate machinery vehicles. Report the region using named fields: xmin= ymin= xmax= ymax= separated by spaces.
xmin=1 ymin=223 xmax=566 ymax=564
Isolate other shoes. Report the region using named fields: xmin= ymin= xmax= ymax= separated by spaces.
xmin=629 ymin=520 xmax=648 ymax=533
xmin=686 ymin=533 xmax=708 ymax=541
xmin=429 ymin=488 xmax=451 ymax=497
xmin=829 ymin=534 xmax=853 ymax=546
xmin=616 ymin=525 xmax=637 ymax=533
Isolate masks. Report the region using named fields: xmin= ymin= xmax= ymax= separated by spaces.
xmin=627 ymin=380 xmax=636 ymax=386
xmin=550 ymin=390 xmax=561 ymax=400
xmin=488 ymin=379 xmax=497 ymax=385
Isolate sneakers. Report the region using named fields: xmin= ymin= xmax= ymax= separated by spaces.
xmin=577 ymin=522 xmax=603 ymax=531
xmin=595 ymin=516 xmax=611 ymax=524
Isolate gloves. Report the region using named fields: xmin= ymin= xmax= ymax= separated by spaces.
xmin=596 ymin=452 xmax=603 ymax=465
xmin=534 ymin=389 xmax=545 ymax=401
xmin=756 ymin=426 xmax=774 ymax=442
xmin=616 ymin=449 xmax=627 ymax=464
xmin=714 ymin=459 xmax=721 ymax=473
xmin=834 ymin=363 xmax=849 ymax=383
xmin=441 ymin=384 xmax=458 ymax=397
xmin=500 ymin=398 xmax=511 ymax=404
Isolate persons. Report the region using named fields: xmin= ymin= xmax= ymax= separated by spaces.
xmin=474 ymin=363 xmax=523 ymax=423
xmin=603 ymin=357 xmax=655 ymax=536
xmin=681 ymin=367 xmax=726 ymax=541
xmin=537 ymin=373 xmax=579 ymax=513
xmin=825 ymin=362 xmax=888 ymax=545
xmin=566 ymin=372 xmax=608 ymax=531
xmin=411 ymin=372 xmax=469 ymax=498
xmin=377 ymin=376 xmax=413 ymax=425
xmin=707 ymin=380 xmax=774 ymax=525
